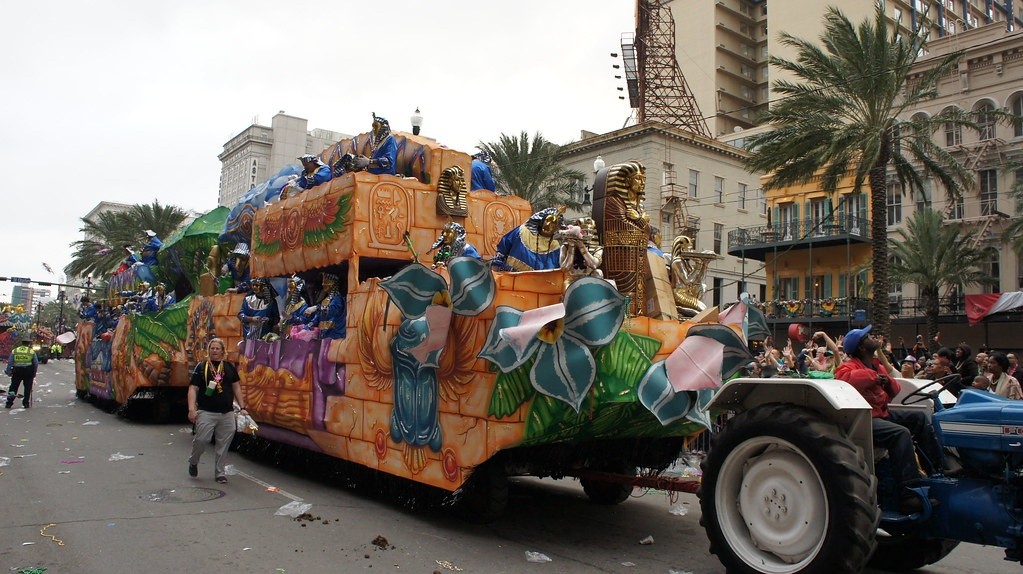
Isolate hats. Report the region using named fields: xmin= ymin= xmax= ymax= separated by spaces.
xmin=824 ymin=350 xmax=833 ymax=357
xmin=22 ymin=339 xmax=33 ymax=346
xmin=843 ymin=324 xmax=873 ymax=354
xmin=900 ymin=355 xmax=917 ymax=366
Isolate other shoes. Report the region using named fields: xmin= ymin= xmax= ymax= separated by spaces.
xmin=189 ymin=464 xmax=198 ymax=476
xmin=24 ymin=404 xmax=29 ymax=408
xmin=215 ymin=476 xmax=227 ymax=483
xmin=5 ymin=404 xmax=11 ymax=408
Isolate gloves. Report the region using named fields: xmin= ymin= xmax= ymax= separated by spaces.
xmin=355 ymin=155 xmax=370 ymax=168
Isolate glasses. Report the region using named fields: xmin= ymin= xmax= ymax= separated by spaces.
xmin=860 ymin=334 xmax=871 ymax=345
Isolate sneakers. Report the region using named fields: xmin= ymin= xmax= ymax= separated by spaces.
xmin=901 ymin=493 xmax=938 ymax=512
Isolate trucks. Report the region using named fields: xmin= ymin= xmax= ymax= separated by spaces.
xmin=75 ymin=131 xmax=1023 ymax=574
xmin=0 ymin=302 xmax=55 ymax=364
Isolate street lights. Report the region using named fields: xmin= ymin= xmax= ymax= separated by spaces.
xmin=80 ymin=277 xmax=97 ymax=297
xmin=34 ymin=301 xmax=44 ymax=327
xmin=56 ymin=291 xmax=69 ymax=335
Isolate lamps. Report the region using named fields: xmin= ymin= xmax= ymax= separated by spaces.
xmin=410 ymin=106 xmax=422 ymax=136
xmin=593 ymin=152 xmax=605 ymax=176
xmin=582 ymin=185 xmax=594 ymax=214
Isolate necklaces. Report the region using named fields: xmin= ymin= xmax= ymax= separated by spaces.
xmin=536 ymin=234 xmax=553 ymax=269
xmin=208 ymin=360 xmax=224 ymax=393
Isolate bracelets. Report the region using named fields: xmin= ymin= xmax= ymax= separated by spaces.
xmin=241 ymin=407 xmax=246 ymax=410
xmin=582 ymin=250 xmax=587 ymax=255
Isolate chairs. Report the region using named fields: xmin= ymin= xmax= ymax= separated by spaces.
xmin=809 ymin=370 xmax=914 ymax=460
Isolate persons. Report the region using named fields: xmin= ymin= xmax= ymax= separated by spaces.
xmin=559 ymin=218 xmax=617 ymax=299
xmin=222 ymin=243 xmax=346 ymax=340
xmin=669 ymin=235 xmax=711 ymax=309
xmin=745 ymin=322 xmax=1023 ymax=508
xmin=492 ymin=207 xmax=565 ymax=273
xmin=299 ymin=116 xmax=396 ymax=188
xmin=646 ymin=226 xmax=670 ymax=269
xmin=428 ymin=218 xmax=481 ymax=261
xmin=608 ymin=160 xmax=662 ymax=317
xmin=78 ymin=229 xmax=175 ymax=320
xmin=188 ymin=338 xmax=248 ymax=484
xmin=50 ymin=343 xmax=62 ymax=360
xmin=4 ymin=339 xmax=38 ymax=408
xmin=470 ymin=150 xmax=497 ymax=192
xmin=437 ymin=164 xmax=470 ymax=217
xmin=748 ymin=294 xmax=757 ymax=304
